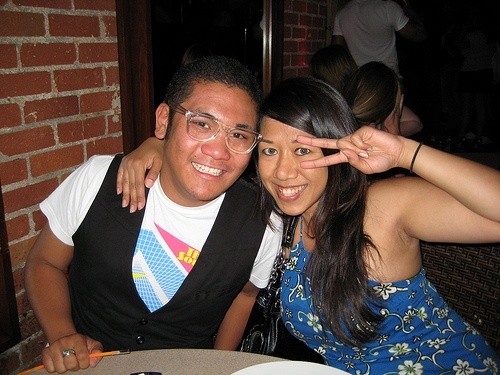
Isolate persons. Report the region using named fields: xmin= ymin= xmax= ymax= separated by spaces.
xmin=454 ymin=14 xmax=494 ymax=144
xmin=330 ymin=0 xmax=428 ymax=77
xmin=342 ymin=61 xmax=404 ymax=135
xmin=22 ymin=55 xmax=283 ymax=373
xmin=310 ymin=44 xmax=423 ymax=137
xmin=117 ymin=76 xmax=500 ymax=375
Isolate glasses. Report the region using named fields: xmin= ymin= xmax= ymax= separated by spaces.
xmin=166 ymin=99 xmax=263 ymax=154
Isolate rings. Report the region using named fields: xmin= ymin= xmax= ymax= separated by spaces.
xmin=62 ymin=349 xmax=75 ymax=357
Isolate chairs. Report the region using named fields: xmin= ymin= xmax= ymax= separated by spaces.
xmin=419 ymin=242 xmax=500 ymax=355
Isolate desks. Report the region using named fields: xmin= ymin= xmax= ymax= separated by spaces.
xmin=27 ymin=349 xmax=290 ymax=375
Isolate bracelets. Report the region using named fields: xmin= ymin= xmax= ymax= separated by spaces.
xmin=410 ymin=143 xmax=424 ymax=173
